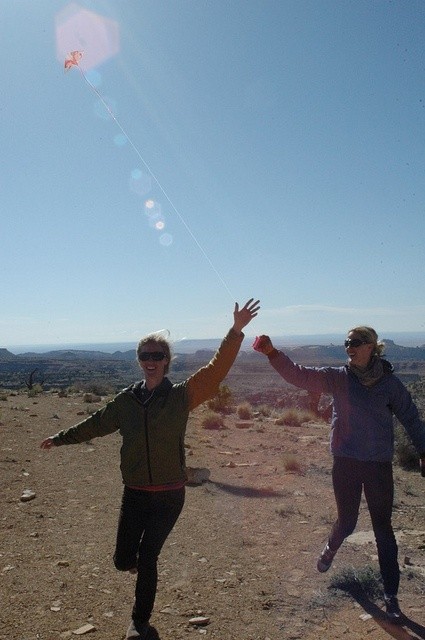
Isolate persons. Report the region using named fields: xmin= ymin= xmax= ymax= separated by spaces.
xmin=40 ymin=298 xmax=261 ymax=639
xmin=252 ymin=326 xmax=425 ymax=622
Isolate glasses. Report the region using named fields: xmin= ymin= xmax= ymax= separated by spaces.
xmin=344 ymin=339 xmax=363 ymax=348
xmin=139 ymin=351 xmax=163 ymax=361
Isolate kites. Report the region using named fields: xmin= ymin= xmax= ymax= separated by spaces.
xmin=64 ymin=48 xmax=88 ymax=73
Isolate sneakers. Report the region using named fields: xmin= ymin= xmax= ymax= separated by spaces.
xmin=384 ymin=595 xmax=401 ymax=623
xmin=317 ymin=543 xmax=336 ymax=572
xmin=127 ymin=619 xmax=149 ymax=639
